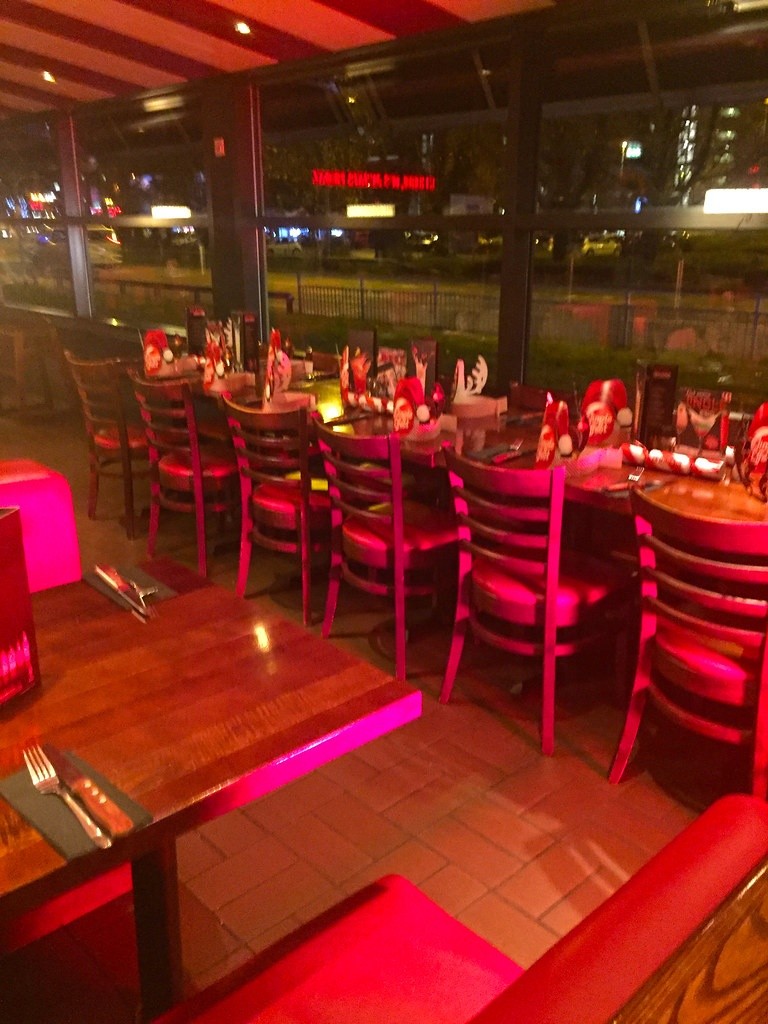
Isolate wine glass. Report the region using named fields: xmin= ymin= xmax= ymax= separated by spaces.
xmin=691 ymin=415 xmax=716 ymax=457
xmin=675 ymin=410 xmax=688 ymax=454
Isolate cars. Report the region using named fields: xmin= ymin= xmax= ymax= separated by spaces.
xmin=579 ymin=234 xmax=623 ymax=258
xmin=83 ymin=224 xmax=121 ymax=269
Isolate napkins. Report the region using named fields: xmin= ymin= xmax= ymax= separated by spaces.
xmin=0 ymin=750 xmax=153 ymax=864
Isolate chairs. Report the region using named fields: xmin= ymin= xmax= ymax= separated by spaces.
xmin=436 ymin=451 xmax=638 ymax=755
xmin=127 ymin=368 xmax=239 ymax=578
xmin=0 ymin=289 xmax=55 ymax=426
xmin=62 ymin=348 xmax=149 ymax=540
xmin=147 ymin=791 xmax=768 ymax=1024
xmin=605 ymin=483 xmax=768 ymax=798
xmin=220 ymin=390 xmax=352 ymax=626
xmin=319 ymin=419 xmax=476 ymax=680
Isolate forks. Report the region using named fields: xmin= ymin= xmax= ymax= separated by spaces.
xmin=119 ymin=575 xmax=158 ymax=598
xmin=20 ymin=743 xmax=112 ymax=849
xmin=627 ymin=466 xmax=644 ymax=482
xmin=487 ymin=438 xmax=524 ymax=457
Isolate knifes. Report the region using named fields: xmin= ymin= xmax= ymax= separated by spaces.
xmin=492 ymin=447 xmax=536 ymax=465
xmin=96 ymin=563 xmax=149 ymax=618
xmin=42 ymin=743 xmax=136 ymax=839
xmin=605 ymin=480 xmax=662 ymax=492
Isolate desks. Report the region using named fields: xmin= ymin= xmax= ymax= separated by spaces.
xmin=63 ymin=353 xmax=768 ymax=540
xmin=0 ymin=555 xmax=424 ymax=1024
